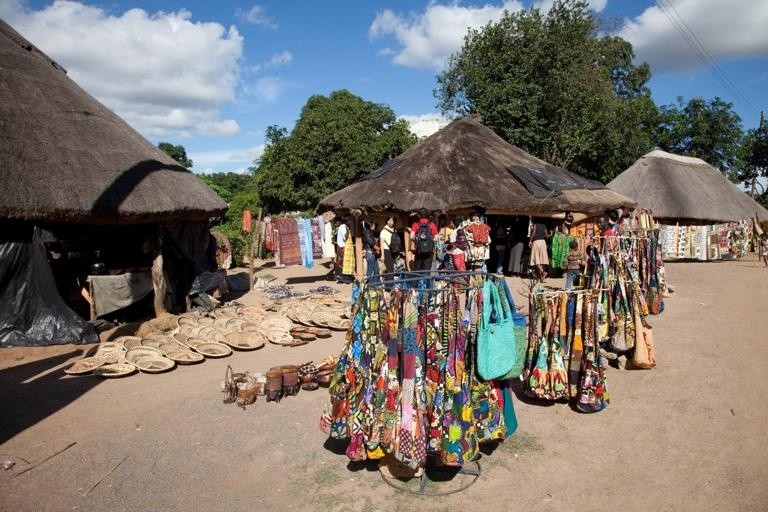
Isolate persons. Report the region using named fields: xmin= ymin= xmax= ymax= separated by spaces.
xmin=585 ymin=245 xmax=599 ymax=274
xmin=494 ymin=214 xmax=557 ymax=282
xmin=363 ymin=211 xmax=465 ymax=287
xmin=566 ymin=241 xmax=581 ymax=286
xmin=597 ymin=214 xmax=614 ymax=253
xmin=760 ymin=233 xmax=768 ymax=267
xmin=563 ymin=212 xmax=575 ymax=230
xmin=330 ymin=214 xmax=354 ymax=283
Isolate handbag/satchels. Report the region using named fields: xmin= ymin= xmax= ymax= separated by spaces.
xmin=523 ymin=291 xmax=611 ymax=413
xmin=580 ymin=210 xmax=676 ymax=369
xmin=320 ymin=275 xmax=529 ymax=470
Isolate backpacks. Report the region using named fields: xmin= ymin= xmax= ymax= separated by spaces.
xmin=383 ymin=227 xmax=402 ymax=255
xmin=414 ymin=219 xmax=435 ymax=257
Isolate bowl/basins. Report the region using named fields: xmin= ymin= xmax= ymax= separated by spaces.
xmin=290 ymin=326 xmax=331 ymax=341
